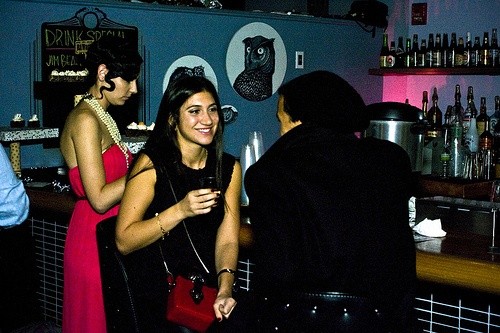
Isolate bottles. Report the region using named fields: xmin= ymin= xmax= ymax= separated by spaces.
xmin=418 ymin=39 xmax=427 ymax=68
xmin=411 ymin=34 xmax=419 ymax=67
xmin=463 ymin=32 xmax=472 ymax=68
xmin=455 ymin=38 xmax=464 ymax=68
xmin=404 ymin=38 xmax=413 ymax=68
xmin=380 ymin=34 xmax=390 ymax=68
xmin=489 ymin=28 xmax=500 ymax=67
xmin=387 ymin=41 xmax=396 ymax=68
xmin=481 ymin=31 xmax=490 ymax=68
xmin=422 ymin=85 xmax=500 ymax=182
xmin=449 ymin=33 xmax=457 ymax=68
xmin=472 ymin=37 xmax=481 ymax=68
xmin=396 ymin=36 xmax=405 ymax=68
xmin=426 ymin=33 xmax=434 ymax=68
xmin=433 ymin=33 xmax=442 ymax=68
xmin=441 ymin=34 xmax=450 ymax=68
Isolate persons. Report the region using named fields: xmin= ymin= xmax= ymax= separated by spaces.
xmin=115 ymin=75 xmax=242 ymax=333
xmin=0 ymin=143 xmax=31 ymax=230
xmin=244 ymin=71 xmax=415 ymax=309
xmin=59 ymin=35 xmax=143 ymax=333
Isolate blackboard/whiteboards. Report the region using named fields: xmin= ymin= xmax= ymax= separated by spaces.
xmin=39 ymin=21 xmax=140 ymax=150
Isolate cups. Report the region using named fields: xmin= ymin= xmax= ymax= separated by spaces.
xmin=238 ymin=130 xmax=266 ymax=206
xmin=199 ymin=176 xmax=217 ymax=204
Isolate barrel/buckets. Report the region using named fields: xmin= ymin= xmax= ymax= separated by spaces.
xmin=365 ymin=103 xmax=424 ymax=173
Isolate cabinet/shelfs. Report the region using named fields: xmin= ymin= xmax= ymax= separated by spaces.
xmin=369 ymin=67 xmax=500 ymax=201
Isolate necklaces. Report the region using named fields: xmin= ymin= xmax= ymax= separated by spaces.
xmin=83 ymin=93 xmax=130 ymax=168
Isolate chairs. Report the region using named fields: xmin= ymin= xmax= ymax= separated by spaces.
xmin=96 ymin=215 xmax=193 ymax=333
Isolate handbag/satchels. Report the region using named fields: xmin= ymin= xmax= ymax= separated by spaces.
xmin=166 ymin=274 xmax=219 ymax=332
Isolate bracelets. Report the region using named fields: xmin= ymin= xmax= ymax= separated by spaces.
xmin=217 ymin=269 xmax=238 ymax=285
xmin=156 ymin=215 xmax=169 ymax=239
